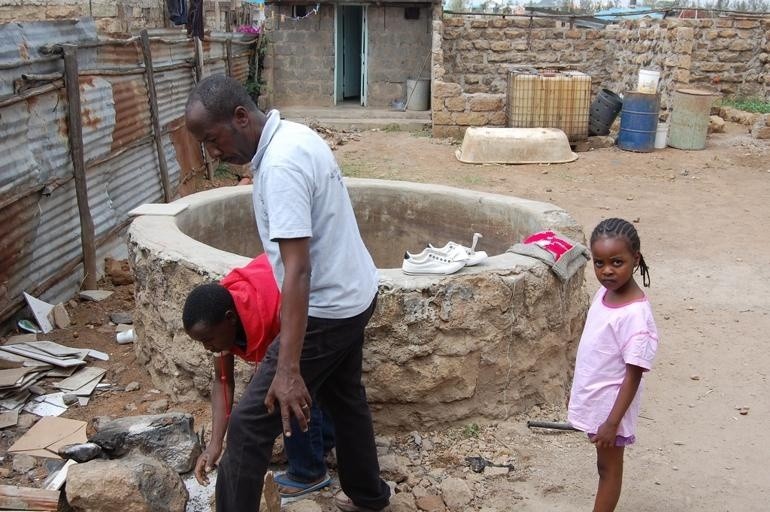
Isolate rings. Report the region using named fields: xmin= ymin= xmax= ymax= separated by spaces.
xmin=301 ymin=404 xmax=308 ymax=410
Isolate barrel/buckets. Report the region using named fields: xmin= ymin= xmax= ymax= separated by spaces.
xmin=618 ymin=90 xmax=661 ymax=153
xmin=638 ymin=69 xmax=660 ymax=94
xmin=669 ymin=89 xmax=713 ymax=150
xmin=654 ymin=122 xmax=668 ymax=150
xmin=406 ymin=78 xmax=430 ymax=110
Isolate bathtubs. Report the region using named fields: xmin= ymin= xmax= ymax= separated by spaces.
xmin=455 ymin=126 xmax=580 ymax=165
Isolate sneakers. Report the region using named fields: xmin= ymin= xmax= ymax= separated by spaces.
xmin=333 ymin=486 xmax=372 ymax=512
xmin=402 ymin=248 xmax=467 ymax=275
xmin=425 ymin=233 xmax=488 ymax=266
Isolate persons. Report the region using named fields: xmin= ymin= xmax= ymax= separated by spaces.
xmin=184 ymin=75 xmax=391 ymax=512
xmin=567 ymin=217 xmax=660 ymax=512
xmin=184 ymin=250 xmax=359 ymax=496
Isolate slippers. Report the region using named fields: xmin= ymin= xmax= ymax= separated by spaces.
xmin=270 ymin=472 xmax=333 ymax=498
